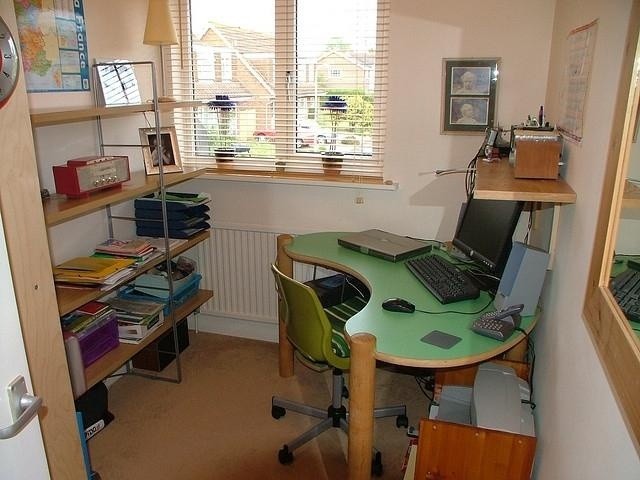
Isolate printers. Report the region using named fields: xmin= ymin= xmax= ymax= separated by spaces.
xmin=436 ymin=362 xmax=536 ymax=438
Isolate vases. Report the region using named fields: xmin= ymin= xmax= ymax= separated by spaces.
xmin=321 ymin=151 xmax=345 ymax=175
xmin=214 ymin=149 xmax=235 ymax=165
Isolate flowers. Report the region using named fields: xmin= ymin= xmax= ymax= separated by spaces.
xmin=319 ymin=94 xmax=349 ymax=155
xmin=209 ymin=95 xmax=236 ymax=147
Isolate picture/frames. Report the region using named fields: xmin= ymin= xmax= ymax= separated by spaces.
xmin=441 ymin=54 xmax=503 ymax=137
xmin=137 ymin=124 xmax=184 ymax=177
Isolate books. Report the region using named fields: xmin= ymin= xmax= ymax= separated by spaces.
xmin=51 ymin=235 xmax=189 ymax=366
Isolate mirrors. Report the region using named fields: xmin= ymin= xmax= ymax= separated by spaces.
xmin=580 ymin=1 xmax=639 ymax=459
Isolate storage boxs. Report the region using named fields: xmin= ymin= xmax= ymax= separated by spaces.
xmin=74 ymin=313 xmax=120 ymax=366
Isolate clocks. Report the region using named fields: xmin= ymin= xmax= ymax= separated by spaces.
xmin=0 ymin=14 xmax=23 ymax=111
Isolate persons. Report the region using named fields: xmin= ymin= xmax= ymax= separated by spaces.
xmin=457 ymin=104 xmax=479 ymax=124
xmin=148 ymin=133 xmax=175 ymax=166
xmin=454 ymin=72 xmax=483 ymax=93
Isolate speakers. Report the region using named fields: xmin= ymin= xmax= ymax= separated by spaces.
xmin=493 ymin=242 xmax=549 ymax=316
xmin=453 ymin=201 xmax=476 ymax=260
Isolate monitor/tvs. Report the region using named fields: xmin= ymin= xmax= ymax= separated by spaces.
xmin=452 ymin=192 xmax=525 ymax=292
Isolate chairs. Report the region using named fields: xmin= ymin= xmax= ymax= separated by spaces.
xmin=266 ymin=264 xmax=410 ymax=474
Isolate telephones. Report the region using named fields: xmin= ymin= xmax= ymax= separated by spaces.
xmin=472 ymin=303 xmax=524 ymax=342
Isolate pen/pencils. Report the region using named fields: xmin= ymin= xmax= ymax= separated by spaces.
xmin=527 ymin=106 xmax=550 ymax=128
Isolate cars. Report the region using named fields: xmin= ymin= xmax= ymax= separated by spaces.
xmin=253 ymin=126 xmax=314 ymax=148
xmin=297 ymin=118 xmax=333 ymax=144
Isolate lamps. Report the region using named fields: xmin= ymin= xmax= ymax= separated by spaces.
xmin=140 ymin=1 xmax=181 ymax=103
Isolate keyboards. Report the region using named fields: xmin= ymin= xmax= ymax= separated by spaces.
xmin=404 ymin=253 xmax=480 ymax=305
xmin=610 ymin=268 xmax=640 ymax=324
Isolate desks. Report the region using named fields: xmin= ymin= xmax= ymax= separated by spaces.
xmin=269 ymin=226 xmax=545 ymax=475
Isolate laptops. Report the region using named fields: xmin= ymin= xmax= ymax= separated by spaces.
xmin=338 ymin=229 xmax=432 ymax=263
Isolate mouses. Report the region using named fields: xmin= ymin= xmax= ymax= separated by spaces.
xmin=382 ymin=298 xmax=414 ymax=313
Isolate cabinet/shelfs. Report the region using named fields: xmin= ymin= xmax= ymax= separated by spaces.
xmin=26 ymin=96 xmax=217 ymax=402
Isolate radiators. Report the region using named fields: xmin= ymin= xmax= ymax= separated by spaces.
xmin=184 ymin=223 xmax=339 ymax=324
